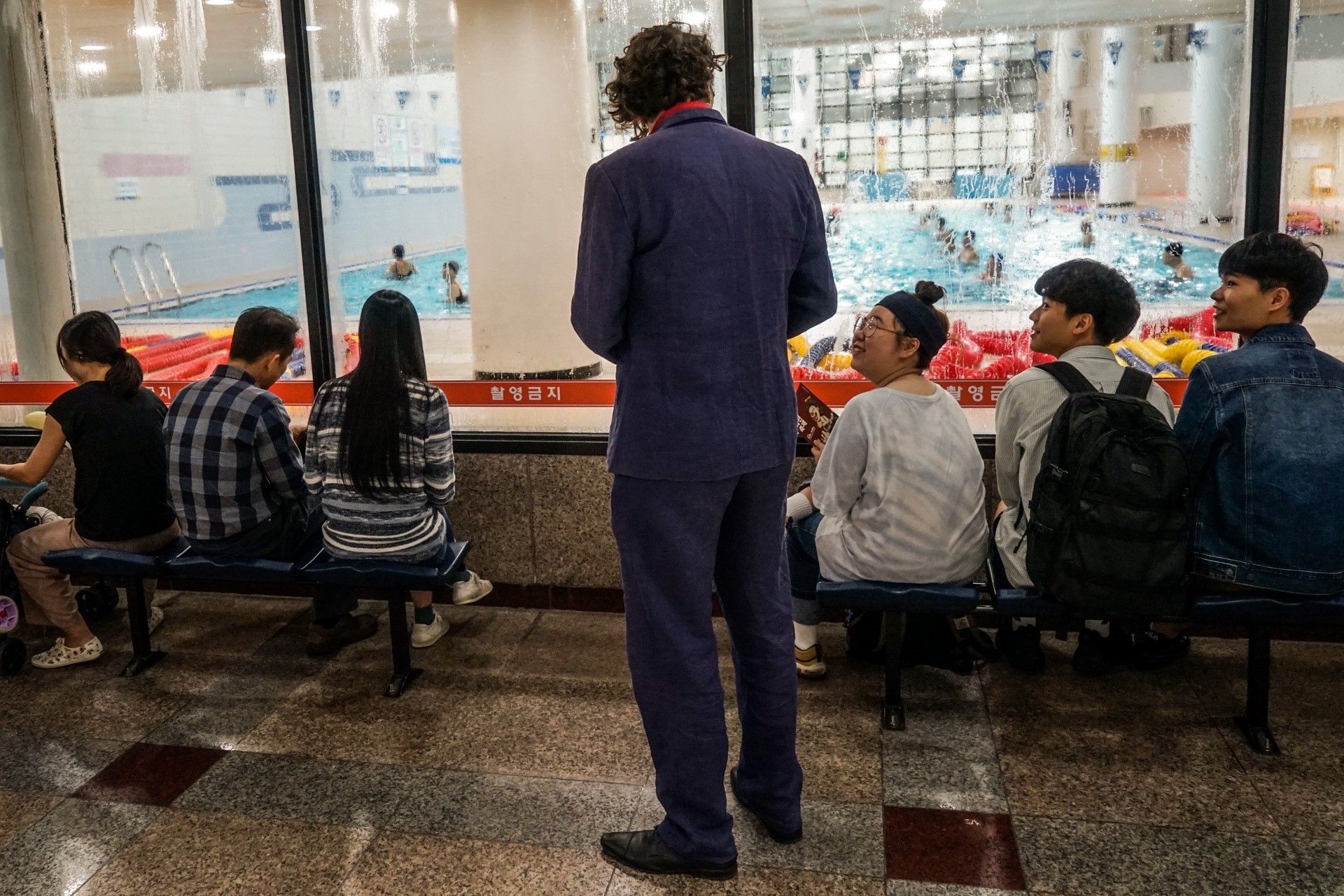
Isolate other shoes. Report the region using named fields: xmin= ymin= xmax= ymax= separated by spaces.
xmin=900 ymin=614 xmax=986 ymax=670
xmin=305 ymin=610 xmax=379 ymax=657
xmin=845 ymin=608 xmax=889 ymax=652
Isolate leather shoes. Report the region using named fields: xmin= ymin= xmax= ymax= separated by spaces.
xmin=599 ymin=825 xmax=738 ymax=880
xmin=730 ymin=765 xmax=803 ymax=845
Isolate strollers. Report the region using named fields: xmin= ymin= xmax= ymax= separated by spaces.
xmin=0 ymin=477 xmax=49 ymax=639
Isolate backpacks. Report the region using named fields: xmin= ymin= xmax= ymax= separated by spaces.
xmin=1011 ymin=361 xmax=1197 ymax=616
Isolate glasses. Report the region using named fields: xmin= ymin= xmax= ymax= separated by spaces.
xmin=853 ymin=316 xmax=900 ymax=338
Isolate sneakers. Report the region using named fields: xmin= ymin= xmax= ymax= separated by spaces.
xmin=1072 ymin=626 xmax=1191 ymax=675
xmin=794 ymin=643 xmax=827 ymax=675
xmin=31 ymin=637 xmax=105 ymax=669
xmin=1009 ymin=624 xmax=1046 ymax=670
xmin=147 ymin=605 xmax=164 ymax=634
xmin=410 ymin=609 xmax=450 ymax=648
xmin=451 ymin=568 xmax=493 ymax=605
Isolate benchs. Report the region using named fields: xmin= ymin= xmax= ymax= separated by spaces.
xmin=815 ymin=560 xmax=1344 ymax=755
xmin=41 ymin=541 xmax=475 ymax=695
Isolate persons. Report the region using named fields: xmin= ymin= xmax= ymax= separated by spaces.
xmin=0 ymin=309 xmax=179 ymax=668
xmin=570 ymin=21 xmax=837 ymax=880
xmin=785 ymin=203 xmax=1344 ymax=676
xmin=384 ymin=244 xmax=418 ymax=280
xmin=303 ymin=289 xmax=493 ymax=647
xmin=163 ymin=306 xmax=377 ymax=657
xmin=441 ymin=260 xmax=468 ymax=304
